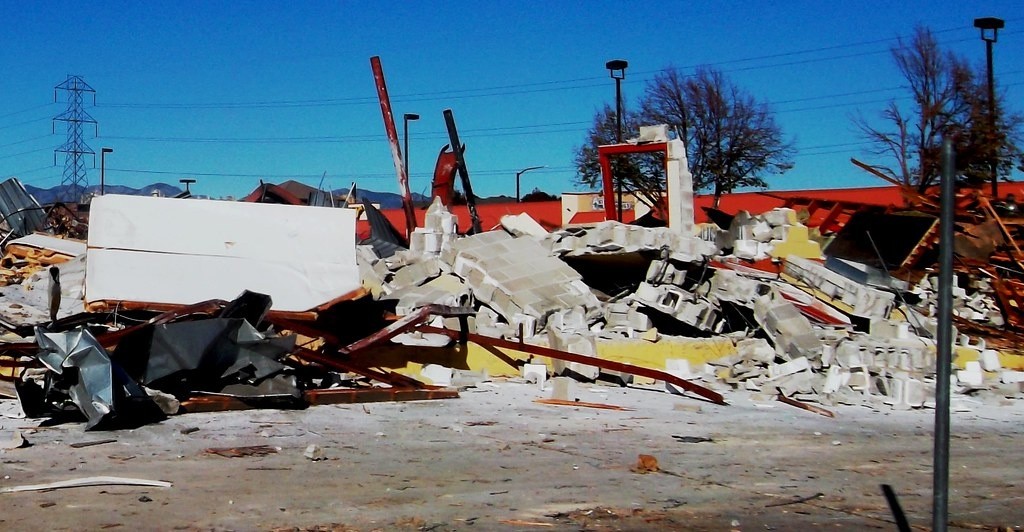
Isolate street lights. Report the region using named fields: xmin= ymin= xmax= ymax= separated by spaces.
xmin=100 ymin=147 xmax=114 ymax=196
xmin=402 ymin=114 xmax=419 ymax=207
xmin=606 ymin=59 xmax=628 ymax=223
xmin=517 ymin=165 xmax=549 ymax=202
xmin=975 ymin=17 xmax=1007 ymax=195
